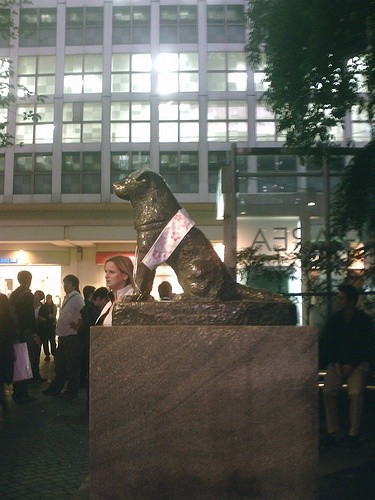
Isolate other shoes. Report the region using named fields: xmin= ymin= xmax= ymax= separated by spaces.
xmin=11 ymin=391 xmax=37 ymax=404
xmin=44 ymin=356 xmax=56 ymax=360
xmin=319 ymin=433 xmax=360 ymax=452
xmin=39 ymin=378 xmax=47 ymax=383
xmin=42 ymin=383 xmax=78 ymax=398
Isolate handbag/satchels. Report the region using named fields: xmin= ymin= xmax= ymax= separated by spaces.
xmin=12 ymin=342 xmax=33 ymax=383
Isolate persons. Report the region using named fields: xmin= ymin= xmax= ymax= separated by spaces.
xmin=158 ymin=281 xmax=172 ymax=301
xmin=95 ymin=255 xmax=140 ymax=326
xmin=0 ymin=270 xmax=109 ymax=402
xmin=318 ymin=283 xmax=375 ymax=446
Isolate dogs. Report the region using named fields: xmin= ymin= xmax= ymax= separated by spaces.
xmin=113 ymin=169 xmax=290 ymax=301
